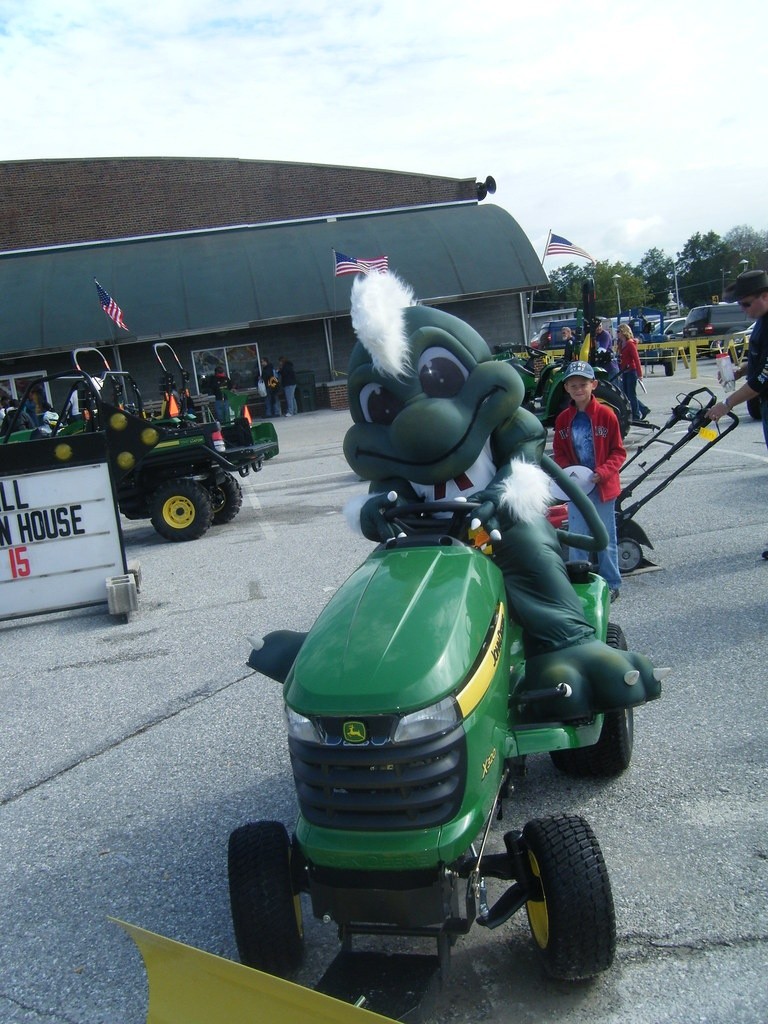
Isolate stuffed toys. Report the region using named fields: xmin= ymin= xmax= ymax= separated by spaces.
xmin=245 ymin=268 xmax=671 ymax=721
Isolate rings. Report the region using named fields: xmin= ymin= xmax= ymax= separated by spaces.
xmin=713 ymin=415 xmax=715 ymax=416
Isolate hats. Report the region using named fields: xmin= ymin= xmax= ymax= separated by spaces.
xmin=562 ymin=361 xmax=595 ymax=384
xmin=723 ymin=269 xmax=768 ymax=303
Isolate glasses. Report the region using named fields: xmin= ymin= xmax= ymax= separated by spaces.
xmin=738 ymin=293 xmax=763 ymax=308
xmin=617 ymin=330 xmax=622 ymax=334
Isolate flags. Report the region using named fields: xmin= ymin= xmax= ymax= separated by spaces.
xmin=335 ymin=252 xmax=389 ymax=278
xmin=545 ymin=233 xmax=596 ymax=266
xmin=96 ymin=281 xmax=128 ymax=331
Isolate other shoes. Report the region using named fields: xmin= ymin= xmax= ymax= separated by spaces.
xmin=608 ymin=587 xmax=620 ymax=603
xmin=285 ymin=413 xmax=292 ymax=417
xmin=262 ymin=414 xmax=272 ymax=418
xmin=641 ymin=408 xmax=652 ymax=420
xmin=632 ymin=417 xmax=640 ymax=422
xmin=273 ymin=413 xmax=282 ymax=417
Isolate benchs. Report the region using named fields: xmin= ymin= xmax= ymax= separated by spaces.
xmin=231 ymin=385 xmax=288 ymax=417
xmin=143 ymin=394 xmax=209 ymax=423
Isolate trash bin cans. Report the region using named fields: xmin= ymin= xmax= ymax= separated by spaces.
xmin=295 ymin=370 xmax=318 ymax=412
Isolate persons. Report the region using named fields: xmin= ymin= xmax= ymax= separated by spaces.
xmin=561 ymin=327 xmax=575 ymax=360
xmin=616 ymin=324 xmax=651 ymax=422
xmin=0 ymin=391 xmax=37 ymax=437
xmin=210 ymin=367 xmax=233 ymax=423
xmin=184 ymin=388 xmax=194 ymax=414
xmin=704 ymin=269 xmax=768 ymax=559
xmin=595 ymin=320 xmax=613 ymax=350
xmin=262 ymin=363 xmax=282 ymax=419
xmin=552 ymin=361 xmax=627 ymax=603
xmin=277 ymin=357 xmax=298 ymax=416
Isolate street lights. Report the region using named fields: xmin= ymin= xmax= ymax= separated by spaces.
xmin=611 ymin=273 xmax=622 ymax=315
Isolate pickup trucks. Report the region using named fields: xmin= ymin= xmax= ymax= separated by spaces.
xmin=0 ymin=339 xmax=280 ymax=544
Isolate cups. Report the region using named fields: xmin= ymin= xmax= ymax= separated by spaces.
xmin=716 ymin=352 xmax=736 ymax=392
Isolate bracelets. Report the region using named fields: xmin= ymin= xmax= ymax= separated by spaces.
xmin=722 ymin=398 xmax=733 ymax=410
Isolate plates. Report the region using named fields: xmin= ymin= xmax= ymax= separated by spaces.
xmin=550 ymin=465 xmax=596 ymax=501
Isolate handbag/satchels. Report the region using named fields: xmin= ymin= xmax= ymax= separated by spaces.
xmin=257 ymin=378 xmax=268 ymax=397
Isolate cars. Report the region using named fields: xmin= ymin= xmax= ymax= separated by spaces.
xmin=530 ymin=300 xmax=758 ymax=363
